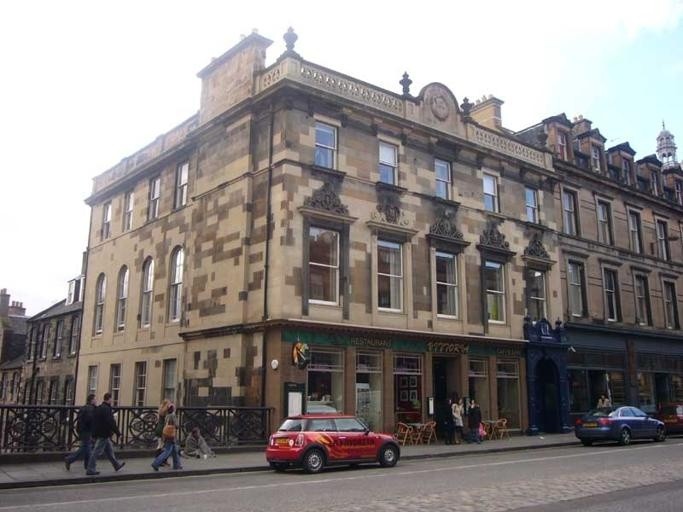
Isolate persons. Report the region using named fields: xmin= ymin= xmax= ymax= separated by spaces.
xmin=442 ymin=398 xmax=454 ymax=445
xmin=149 ymin=403 xmax=184 ymax=472
xmin=594 ymin=392 xmax=612 ymax=409
xmin=151 ymin=397 xmax=173 ymax=467
xmin=64 ymin=394 xmax=98 ymax=473
xmin=467 ymin=399 xmax=481 ymax=445
xmin=85 ymin=391 xmax=125 ymax=477
xmin=450 ymin=397 xmax=465 ymax=444
xmin=183 ymin=425 xmax=213 ymax=459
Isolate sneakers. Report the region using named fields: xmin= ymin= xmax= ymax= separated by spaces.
xmin=86 ymin=469 xmax=99 ymax=474
xmin=151 ymin=461 xmax=170 ymax=471
xmin=64 ymin=455 xmax=70 ymax=470
xmin=173 ymin=465 xmax=184 ymax=470
xmin=114 ymin=462 xmax=126 ymax=471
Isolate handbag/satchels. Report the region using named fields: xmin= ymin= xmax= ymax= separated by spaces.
xmin=163 ymin=424 xmax=177 ymax=439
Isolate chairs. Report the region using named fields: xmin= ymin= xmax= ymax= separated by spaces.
xmin=481 ymin=418 xmax=510 ymax=440
xmin=396 ymin=421 xmax=438 ymax=446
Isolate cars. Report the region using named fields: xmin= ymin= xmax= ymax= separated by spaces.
xmin=264 ymin=413 xmax=404 ymax=472
xmin=574 ymin=405 xmax=668 ymax=447
xmin=652 ymin=402 xmax=682 ymax=435
